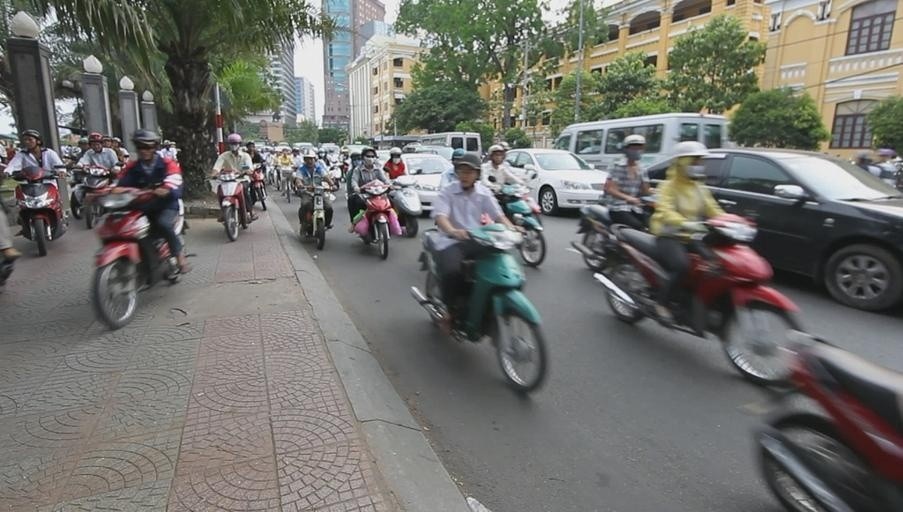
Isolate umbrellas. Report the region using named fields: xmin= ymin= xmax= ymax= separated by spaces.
xmin=879 ymin=149 xmax=897 ymax=156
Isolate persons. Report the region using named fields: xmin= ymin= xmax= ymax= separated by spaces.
xmin=857 ymin=155 xmax=869 ymax=171
xmin=649 ymin=138 xmax=726 ymax=323
xmin=431 ymin=144 xmax=534 ymax=325
xmin=602 ymin=134 xmax=653 ymax=231
xmin=210 ymin=134 xmax=408 ymax=232
xmin=0 ymin=127 xmax=192 ymax=282
xmin=879 ymin=155 xmax=888 ymax=162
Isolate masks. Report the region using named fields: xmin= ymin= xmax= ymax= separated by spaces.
xmin=353 ymin=159 xmax=362 ymax=167
xmin=228 ymin=143 xmax=239 ymax=151
xmin=625 ymin=150 xmax=643 ymax=160
xmin=364 ymin=156 xmax=376 ymax=164
xmin=688 ymin=165 xmax=707 ymax=177
xmin=392 ymin=158 xmax=400 ymax=165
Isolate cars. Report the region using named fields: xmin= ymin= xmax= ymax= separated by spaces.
xmin=375 ymin=131 xmax=480 ymax=215
xmin=239 ymin=139 xmax=373 ymax=162
xmin=503 ymin=147 xmax=610 ymax=213
xmin=643 ymin=148 xmax=903 ymax=314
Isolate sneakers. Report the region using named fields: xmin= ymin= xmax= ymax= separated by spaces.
xmin=218 ymin=215 xmax=225 ymax=222
xmin=2 ymin=249 xmax=22 ymax=262
xmin=250 ymin=212 xmax=258 ymax=220
xmin=178 ymin=256 xmax=191 ymax=273
xmin=348 ymin=221 xmax=354 ymax=233
xmin=654 ymin=304 xmax=675 ymax=322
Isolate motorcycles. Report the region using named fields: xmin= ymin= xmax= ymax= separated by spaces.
xmin=211 ymin=163 xmax=423 ymax=262
xmin=593 ymin=203 xmax=807 ymax=389
xmin=411 ymin=210 xmax=549 ymax=397
xmin=568 ymin=190 xmax=738 ymax=275
xmin=0 ymin=153 xmax=130 ymax=286
xmin=746 ymin=321 xmax=902 ymax=512
xmin=86 ymin=184 xmax=187 ymax=331
xmin=486 ymin=170 xmax=545 ymax=269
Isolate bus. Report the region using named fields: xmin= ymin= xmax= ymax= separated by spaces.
xmin=553 ymin=111 xmax=727 ymax=176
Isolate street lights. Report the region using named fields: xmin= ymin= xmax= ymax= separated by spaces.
xmin=117 ymin=76 xmax=140 ymax=156
xmin=80 ymin=55 xmax=114 ymax=138
xmin=139 ymin=89 xmax=156 ymax=132
xmin=4 ymin=10 xmax=72 ymax=221
xmin=61 ymin=78 xmax=83 ymax=138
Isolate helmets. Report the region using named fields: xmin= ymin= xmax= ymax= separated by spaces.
xmin=351 ymin=146 xmax=402 ymax=160
xmin=856 ymin=149 xmax=897 ymax=161
xmin=131 ymin=128 xmax=176 ymax=147
xmin=22 ymin=129 xmax=43 ymax=146
xmin=228 ymin=133 xmax=242 ymax=143
xmin=673 ymin=141 xmax=709 ymax=157
xmin=622 ymin=134 xmax=646 ymax=147
xmin=78 ymin=133 xmax=121 ymax=144
xmin=246 ymin=140 xmax=255 ymax=145
xmin=274 ymin=141 xmax=348 ymax=157
xmin=452 ymin=141 xmax=511 ymax=170
